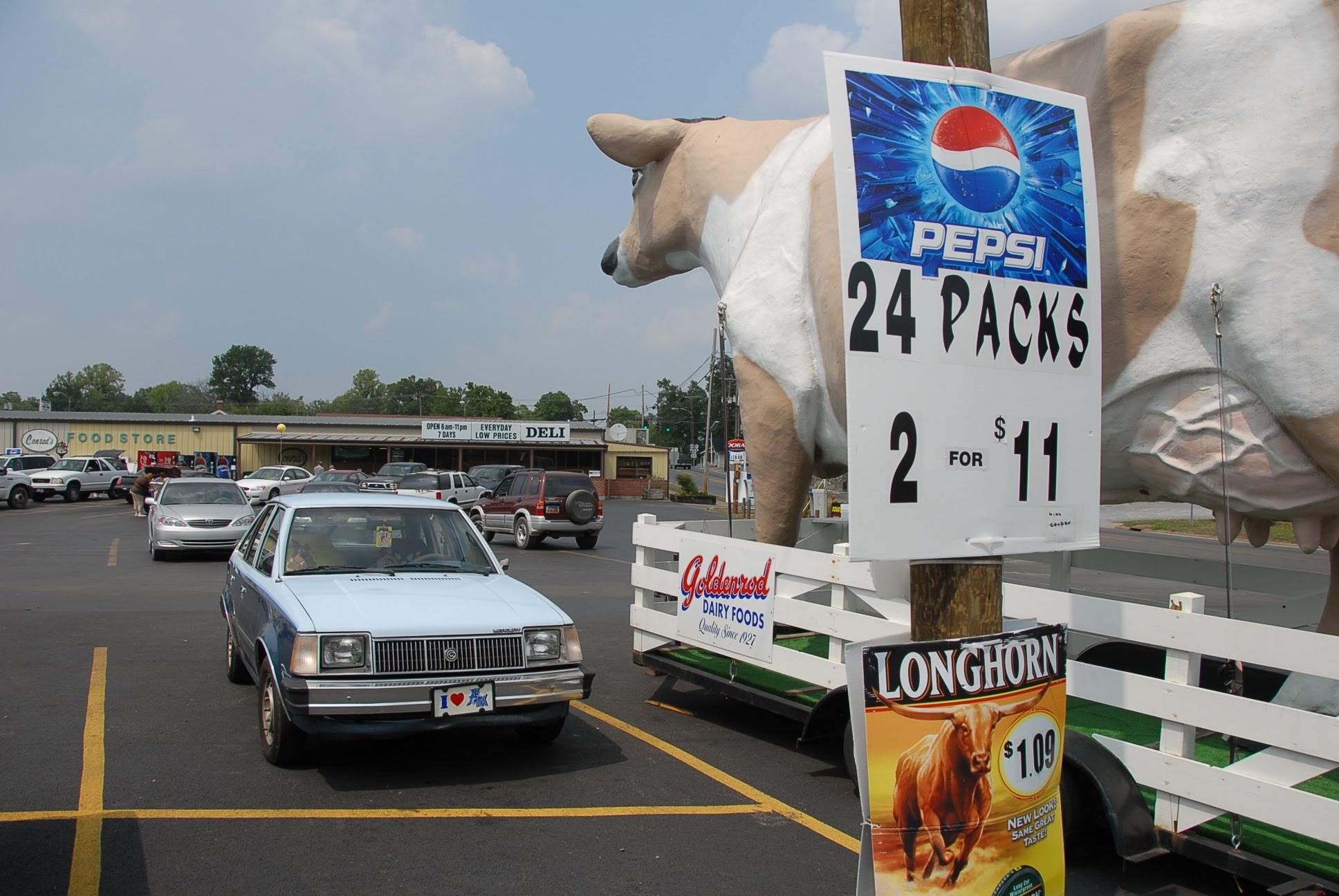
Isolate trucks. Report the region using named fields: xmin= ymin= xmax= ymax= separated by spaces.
xmin=631 ymin=487 xmax=1339 ymax=896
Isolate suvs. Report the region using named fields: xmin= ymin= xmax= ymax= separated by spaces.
xmin=465 ymin=469 xmax=603 ymax=550
xmin=0 ymin=453 xmax=57 ymax=509
xmin=395 ymin=469 xmax=493 ymax=528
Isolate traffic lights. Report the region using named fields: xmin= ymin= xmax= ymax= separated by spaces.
xmin=644 ymin=426 xmax=647 ymax=429
xmin=667 ymin=428 xmax=670 ymax=431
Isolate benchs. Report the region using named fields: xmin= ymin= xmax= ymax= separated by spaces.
xmin=336 ymin=543 xmax=384 ymax=567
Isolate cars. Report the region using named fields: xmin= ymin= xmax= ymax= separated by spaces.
xmin=235 ymin=465 xmax=316 ymax=505
xmin=220 ymin=492 xmax=596 ymax=766
xmin=296 ymin=470 xmax=372 ymax=493
xmin=113 ymin=463 xmax=220 ymax=514
xmin=463 ymin=465 xmax=526 ymax=496
xmin=145 ymin=477 xmax=256 ymax=561
xmin=358 ymin=462 xmax=433 ymax=493
xmin=291 ymin=481 xmax=367 ymax=543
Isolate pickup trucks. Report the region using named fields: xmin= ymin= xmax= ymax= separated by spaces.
xmin=670 ymin=453 xmax=692 ymax=469
xmin=26 ymin=449 xmax=129 ymax=503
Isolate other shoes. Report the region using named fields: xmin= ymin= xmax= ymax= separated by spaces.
xmin=138 ymin=514 xmax=147 ymax=517
xmin=135 ymin=513 xmax=138 ymax=517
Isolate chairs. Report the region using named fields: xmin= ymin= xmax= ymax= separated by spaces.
xmin=380 ymin=536 xmax=433 ymax=563
xmin=265 ymin=530 xmax=347 ymax=568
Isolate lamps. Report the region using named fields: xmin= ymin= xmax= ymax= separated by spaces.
xmin=192 ymin=426 xmax=200 ymax=432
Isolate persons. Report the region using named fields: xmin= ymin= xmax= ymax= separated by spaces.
xmin=314 ymin=461 xmax=324 ymax=476
xmin=329 ymin=465 xmax=336 ymax=470
xmin=130 ymin=473 xmax=153 ymax=517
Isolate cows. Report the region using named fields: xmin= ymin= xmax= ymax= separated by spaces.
xmin=584 ymin=0 xmax=1338 ymax=633
xmin=870 ymin=674 xmax=1055 ymax=887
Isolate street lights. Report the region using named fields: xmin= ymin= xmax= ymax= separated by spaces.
xmin=52 ymin=392 xmax=71 ymax=412
xmin=276 ymin=424 xmax=286 ymax=465
xmin=671 ymin=408 xmax=695 ymax=467
